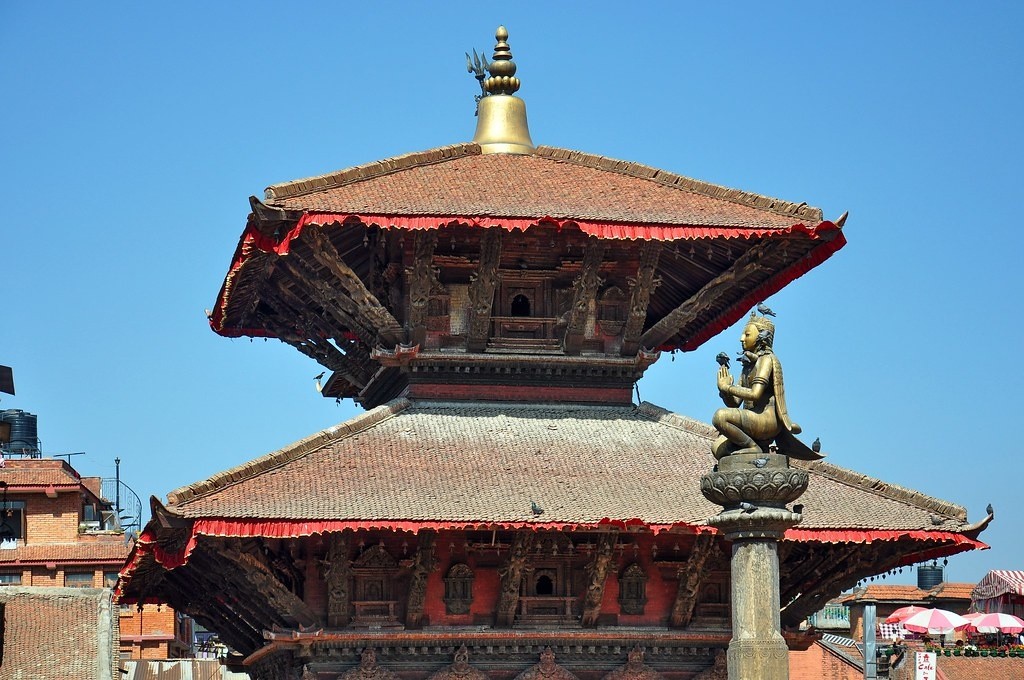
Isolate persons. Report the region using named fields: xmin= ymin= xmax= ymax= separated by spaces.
xmin=710 ymin=316 xmax=828 ymax=461
xmin=1010 ymin=632 xmax=1024 ymax=645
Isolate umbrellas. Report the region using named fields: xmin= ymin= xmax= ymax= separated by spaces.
xmin=954 ymin=612 xmax=984 ymax=641
xmin=898 ymin=607 xmax=972 ymax=643
xmin=885 ymin=605 xmax=929 ymax=624
xmin=971 ymin=613 xmax=1024 ymax=648
